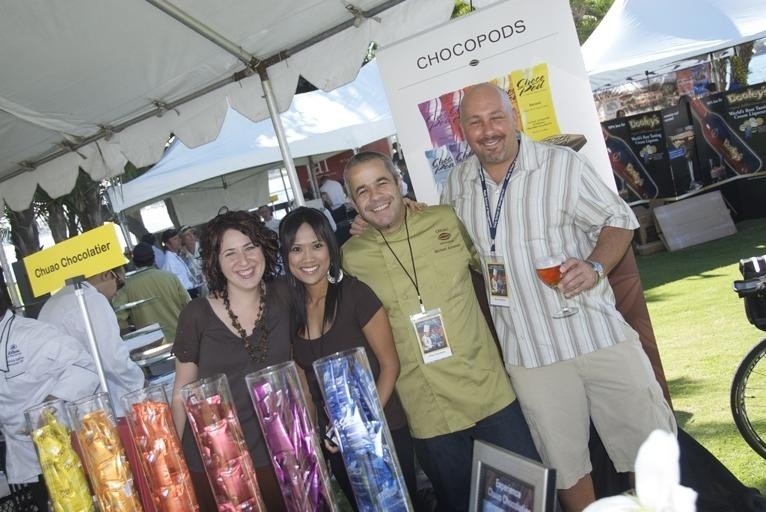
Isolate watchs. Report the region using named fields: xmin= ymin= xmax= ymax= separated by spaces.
xmin=584 ymin=260 xmax=603 ymax=285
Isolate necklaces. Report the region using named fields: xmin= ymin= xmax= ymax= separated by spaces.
xmin=220 ymin=282 xmax=270 ymax=368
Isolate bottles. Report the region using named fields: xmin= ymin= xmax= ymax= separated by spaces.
xmin=691 ymin=99 xmax=762 ymax=176
xmin=601 ymin=124 xmax=659 ymax=201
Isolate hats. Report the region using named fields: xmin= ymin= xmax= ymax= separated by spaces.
xmin=133 ymin=243 xmax=153 ymax=262
xmin=163 ymin=226 xmax=198 ymax=242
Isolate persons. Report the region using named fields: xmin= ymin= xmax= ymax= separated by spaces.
xmin=349 ymin=83 xmax=678 ymax=512
xmin=170 ymin=197 xmax=428 ymax=512
xmin=278 ymin=207 xmax=418 ymax=512
xmin=38 ymin=266 xmax=149 ymax=417
xmin=339 ymin=151 xmax=543 ymax=512
xmin=144 ymin=176 xmax=360 ymax=302
xmin=111 ymin=244 xmax=192 ymax=344
xmin=0 ymin=267 xmax=100 ymax=512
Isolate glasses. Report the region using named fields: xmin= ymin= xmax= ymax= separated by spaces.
xmin=109 ymin=269 xmax=126 ymax=291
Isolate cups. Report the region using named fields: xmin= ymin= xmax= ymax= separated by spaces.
xmin=708 ymin=156 xmax=727 ymax=183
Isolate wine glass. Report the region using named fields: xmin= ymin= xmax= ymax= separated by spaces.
xmin=669 ymin=125 xmax=705 ymax=192
xmin=527 ymin=237 xmax=580 ymax=318
